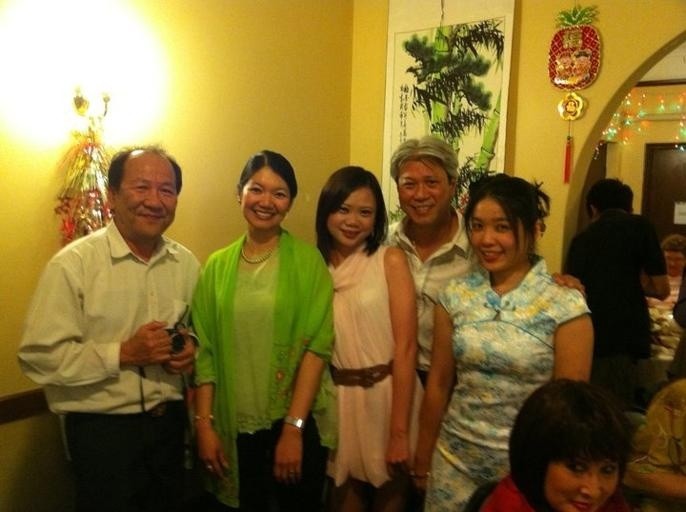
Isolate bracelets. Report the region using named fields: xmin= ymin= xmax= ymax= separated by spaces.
xmin=193 ymin=413 xmax=215 ymax=425
xmin=284 ymin=416 xmax=307 ymax=430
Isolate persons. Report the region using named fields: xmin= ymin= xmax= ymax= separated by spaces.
xmin=18 ymin=145 xmax=201 ymax=511
xmin=645 ymin=234 xmax=686 ymax=311
xmin=621 ymin=380 xmax=686 ymax=512
xmin=478 ymin=377 xmax=632 ymax=512
xmin=568 ymin=178 xmax=669 ymax=358
xmin=413 ymin=173 xmax=595 ymax=512
xmin=382 ymin=135 xmax=470 ymax=388
xmin=314 ymin=166 xmax=424 ymax=511
xmin=187 ymin=150 xmax=334 ymax=511
xmin=666 ymin=265 xmax=686 ymax=383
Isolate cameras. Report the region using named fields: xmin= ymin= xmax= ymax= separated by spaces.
xmin=164 ymin=328 xmax=185 ymax=354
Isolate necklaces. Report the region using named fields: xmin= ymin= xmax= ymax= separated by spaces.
xmin=240 ymin=239 xmax=279 ymax=263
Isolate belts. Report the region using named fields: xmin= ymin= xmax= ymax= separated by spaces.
xmin=150 ymin=403 xmax=170 ymax=416
